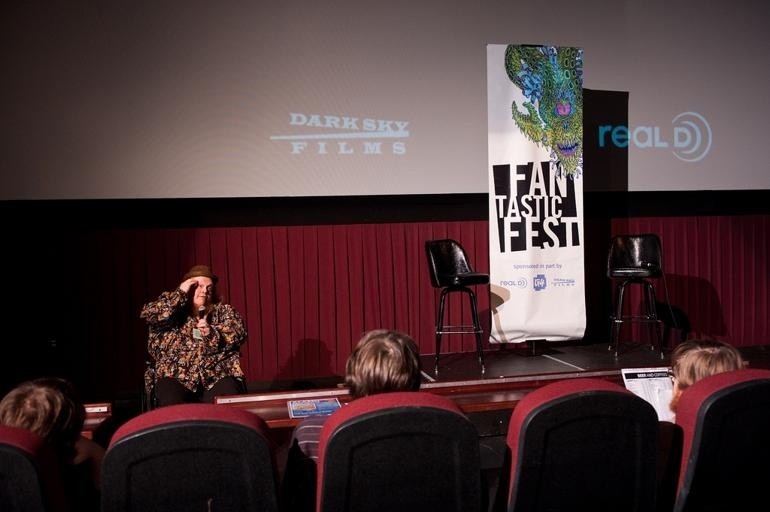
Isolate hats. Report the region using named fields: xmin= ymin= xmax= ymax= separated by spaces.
xmin=181 ymin=265 xmax=217 ymax=285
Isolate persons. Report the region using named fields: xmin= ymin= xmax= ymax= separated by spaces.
xmin=139 ymin=263 xmax=247 ymax=409
xmin=1 ymin=375 xmax=105 ymax=471
xmin=278 ymin=328 xmax=429 ymax=512
xmin=663 ymin=336 xmax=746 ymax=417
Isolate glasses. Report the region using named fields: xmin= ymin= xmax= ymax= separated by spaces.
xmin=667 ymin=369 xmax=680 ymax=383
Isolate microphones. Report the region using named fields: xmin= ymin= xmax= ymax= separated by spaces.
xmin=199 ymin=306 xmax=206 ymax=336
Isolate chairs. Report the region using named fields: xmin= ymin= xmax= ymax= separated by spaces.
xmin=424 ymin=239 xmax=493 ymax=378
xmin=604 ymin=231 xmax=664 ymax=365
